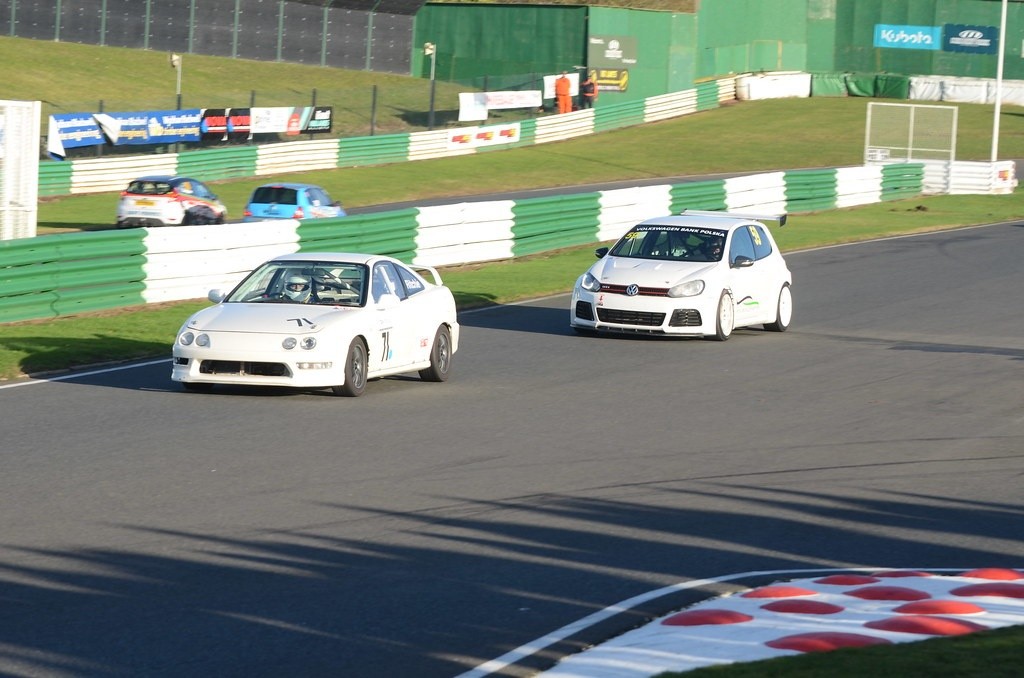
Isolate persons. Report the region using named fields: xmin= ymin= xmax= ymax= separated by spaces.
xmin=581 ymin=75 xmax=596 ymax=108
xmin=555 ymin=71 xmax=572 ymax=114
xmin=704 ymin=236 xmax=723 ymax=260
xmin=283 ymin=268 xmax=321 ymax=303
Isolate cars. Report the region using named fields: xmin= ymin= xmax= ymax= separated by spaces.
xmin=243 ymin=181 xmax=346 ymax=221
xmin=171 ymin=248 xmax=461 ymax=396
xmin=118 ymin=175 xmax=229 ymax=226
xmin=570 ymin=214 xmax=791 ymax=337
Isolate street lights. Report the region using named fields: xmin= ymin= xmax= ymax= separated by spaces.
xmin=171 ymin=55 xmax=181 ymax=112
xmin=425 ymin=43 xmax=435 ymax=129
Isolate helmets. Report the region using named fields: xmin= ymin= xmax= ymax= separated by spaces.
xmin=284 ymin=271 xmax=312 ymax=303
xmin=706 ymin=236 xmax=725 ymax=261
